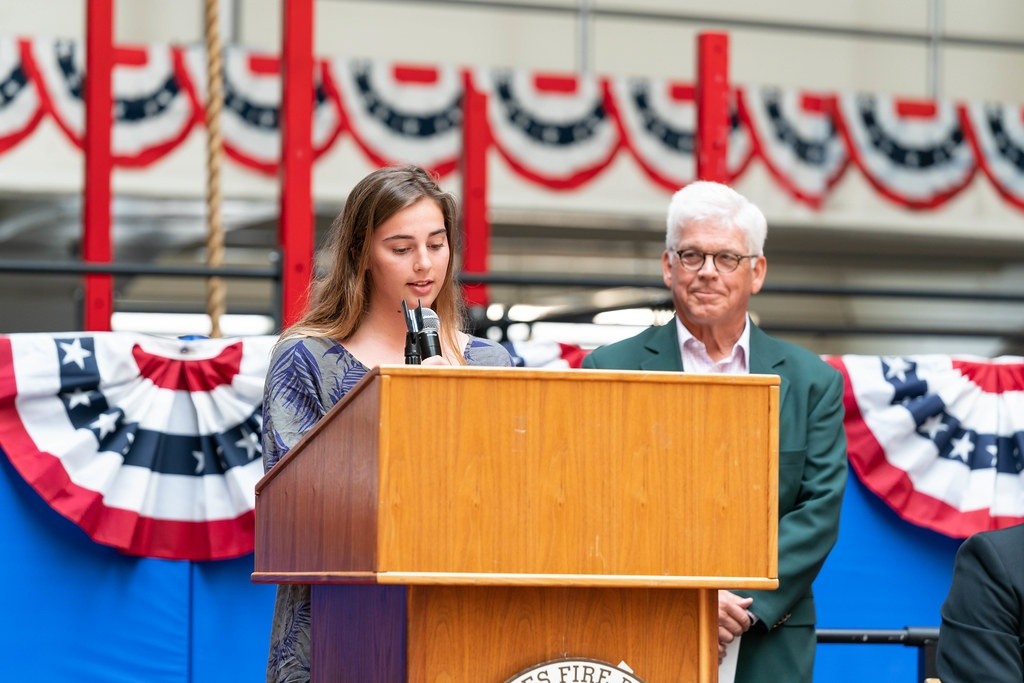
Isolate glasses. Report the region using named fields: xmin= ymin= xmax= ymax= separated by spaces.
xmin=669 ymin=248 xmax=759 ymax=273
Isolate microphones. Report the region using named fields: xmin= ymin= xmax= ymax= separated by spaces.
xmin=417 ymin=308 xmax=442 ymax=361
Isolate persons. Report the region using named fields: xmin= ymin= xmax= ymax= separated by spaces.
xmin=935 ymin=522 xmax=1024 ymax=683
xmin=263 ymin=166 xmax=512 ymax=683
xmin=582 ymin=182 xmax=848 ymax=683
